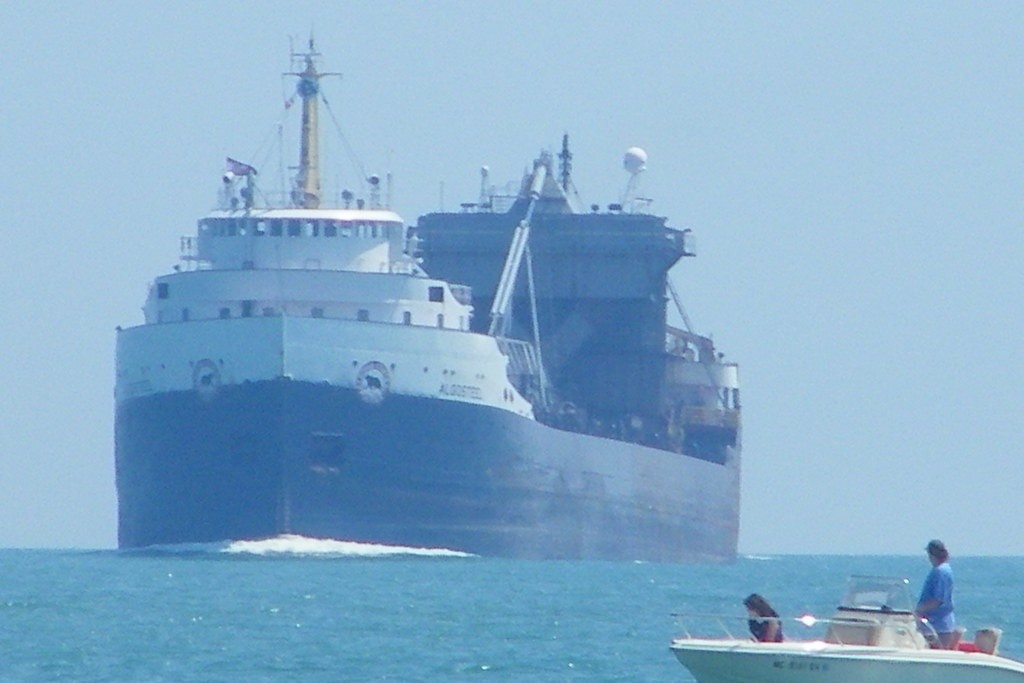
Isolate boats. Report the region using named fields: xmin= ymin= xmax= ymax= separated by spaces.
xmin=666 ymin=574 xmax=1024 ymax=683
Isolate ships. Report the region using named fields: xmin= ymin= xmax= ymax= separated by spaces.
xmin=112 ymin=18 xmax=744 ymax=567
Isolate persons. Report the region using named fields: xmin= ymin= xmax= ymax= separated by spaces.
xmin=914 ymin=539 xmax=956 ymax=646
xmin=743 ymin=593 xmax=783 ymax=642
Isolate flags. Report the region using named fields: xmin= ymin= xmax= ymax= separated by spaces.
xmin=227 ymin=159 xmax=257 ymax=176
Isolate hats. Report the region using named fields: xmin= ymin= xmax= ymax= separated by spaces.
xmin=924 ymin=539 xmax=943 ymax=551
